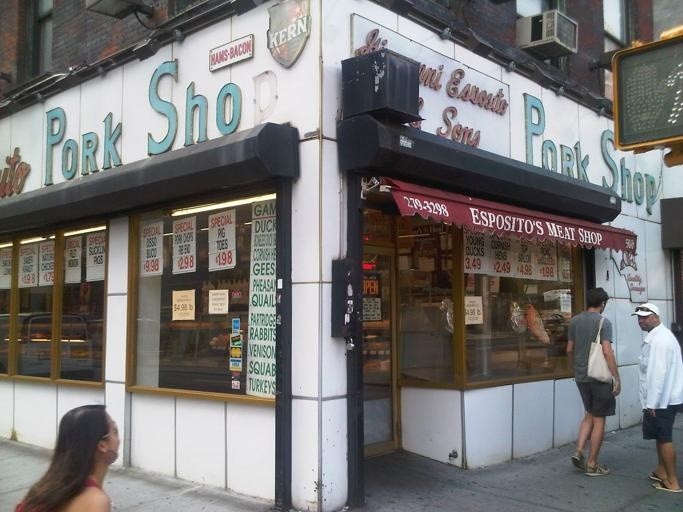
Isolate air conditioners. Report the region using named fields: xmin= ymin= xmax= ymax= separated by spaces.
xmin=543 ymin=10 xmax=580 ymax=54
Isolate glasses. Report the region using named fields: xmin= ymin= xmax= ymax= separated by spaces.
xmin=635 ymin=306 xmax=657 ymax=314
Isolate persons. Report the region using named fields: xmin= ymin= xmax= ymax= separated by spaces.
xmin=630 ymin=303 xmax=682 ymax=494
xmin=12 ymin=404 xmax=120 ymax=511
xmin=564 ymin=288 xmax=622 ymax=477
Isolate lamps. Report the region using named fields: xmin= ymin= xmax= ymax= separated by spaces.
xmin=82 ymin=0 xmax=156 ymax=24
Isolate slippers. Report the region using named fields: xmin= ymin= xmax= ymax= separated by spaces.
xmin=651 ymin=481 xmax=683 ymax=493
xmin=648 ymin=472 xmax=665 ymax=482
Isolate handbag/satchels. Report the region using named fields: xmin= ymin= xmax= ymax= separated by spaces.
xmin=587 ymin=317 xmax=615 ymax=385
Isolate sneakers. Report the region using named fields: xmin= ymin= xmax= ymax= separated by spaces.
xmin=572 ymin=450 xmax=585 ymax=470
xmin=583 ymin=464 xmax=610 ymax=477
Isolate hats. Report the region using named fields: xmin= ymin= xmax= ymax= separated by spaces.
xmin=630 ymin=303 xmax=661 ymax=317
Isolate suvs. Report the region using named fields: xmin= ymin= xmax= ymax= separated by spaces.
xmin=537 ymin=290 xmax=571 ymax=321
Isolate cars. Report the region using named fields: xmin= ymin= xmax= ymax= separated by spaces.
xmin=138 ymin=316 xmax=159 ymax=361
xmin=227 ymin=301 xmax=249 ymax=328
xmin=413 ymin=295 xmax=451 ymax=312
xmin=85 ymin=316 xmax=105 ymax=368
xmin=158 ymin=306 xmax=220 ymax=345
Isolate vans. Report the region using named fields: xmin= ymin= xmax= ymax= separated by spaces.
xmin=0 ymin=312 xmax=95 ymax=379
